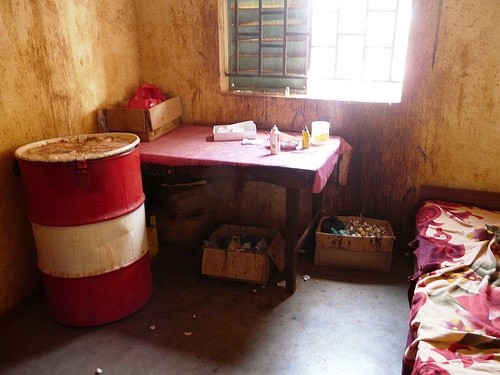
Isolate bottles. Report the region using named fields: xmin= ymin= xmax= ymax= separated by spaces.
xmin=270 ymin=125 xmax=280 ymax=154
xmin=302 ymin=124 xmax=310 ymax=148
xmin=97 ymin=110 xmax=109 ymax=133
xmin=227 ymin=236 xmax=240 ymax=252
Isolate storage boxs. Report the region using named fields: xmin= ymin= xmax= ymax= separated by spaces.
xmin=194 ymin=223 xmax=285 ymax=286
xmin=212 ymin=125 xmax=257 ymax=141
xmin=313 ymin=216 xmax=396 ymax=275
xmin=106 ymin=95 xmax=183 ymax=142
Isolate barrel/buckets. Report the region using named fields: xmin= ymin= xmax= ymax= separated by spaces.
xmin=15 ymin=133 xmax=154 ymax=325
xmin=311 ymin=121 xmax=330 ymax=145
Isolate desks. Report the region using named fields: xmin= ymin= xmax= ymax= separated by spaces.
xmin=140 ymin=124 xmax=352 ymax=295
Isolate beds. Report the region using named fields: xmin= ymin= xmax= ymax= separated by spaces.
xmin=401 ymin=184 xmax=500 ymax=375
